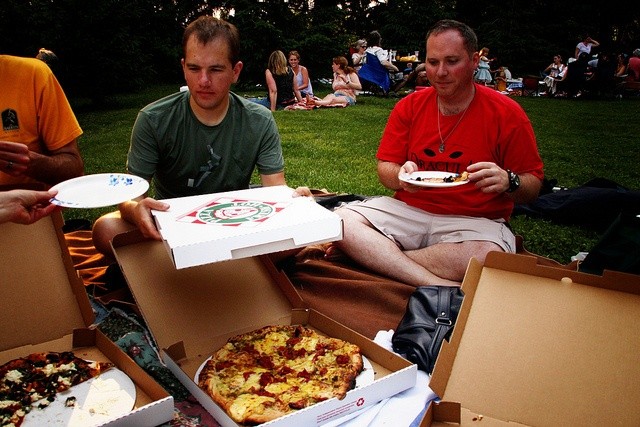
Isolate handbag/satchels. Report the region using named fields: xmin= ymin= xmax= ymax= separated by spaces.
xmin=392 ymin=285 xmax=464 ymax=372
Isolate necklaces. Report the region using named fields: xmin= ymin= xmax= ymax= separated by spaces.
xmin=432 ymin=95 xmax=473 ymax=153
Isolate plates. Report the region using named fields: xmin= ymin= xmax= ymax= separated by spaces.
xmin=399 ymin=170 xmax=471 ymax=187
xmin=48 ymin=172 xmax=150 ymax=208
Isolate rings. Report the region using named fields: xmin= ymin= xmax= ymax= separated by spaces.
xmin=7 ymin=160 xmax=14 ymax=169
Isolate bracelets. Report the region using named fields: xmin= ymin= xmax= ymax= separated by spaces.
xmin=346 ymin=80 xmax=349 ymax=84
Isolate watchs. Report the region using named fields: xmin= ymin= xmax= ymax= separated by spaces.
xmin=505 ymin=168 xmax=521 ymax=193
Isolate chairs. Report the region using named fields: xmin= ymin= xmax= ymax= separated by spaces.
xmin=359 ymin=51 xmax=392 ymax=98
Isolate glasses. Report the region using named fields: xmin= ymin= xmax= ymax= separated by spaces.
xmin=360 ymin=46 xmax=367 ymax=48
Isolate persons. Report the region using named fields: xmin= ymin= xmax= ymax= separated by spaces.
xmin=1 ymin=52 xmax=84 ymax=190
xmin=253 ymin=47 xmax=306 ymax=112
xmin=493 ymin=70 xmax=508 ymax=92
xmin=35 ymin=47 xmax=58 ymax=68
xmin=329 ymin=18 xmax=546 ymax=287
xmin=89 ymin=14 xmax=319 ymax=303
xmin=357 ymin=30 xmax=400 ymax=97
xmin=553 ymin=51 xmax=590 ymax=82
xmin=304 ymin=55 xmax=363 ymax=107
xmin=474 ymin=46 xmax=497 ymax=86
xmin=288 ymin=49 xmax=315 ymax=103
xmin=627 ymin=47 xmax=640 ymax=82
xmin=574 ymin=35 xmax=600 ymax=60
xmin=393 ymin=61 xmax=429 ymax=93
xmin=538 ymin=54 xmax=566 ymax=98
xmin=351 ymin=38 xmax=369 ymax=73
xmin=0 ymin=187 xmax=59 ymax=225
xmin=612 ymin=52 xmax=630 ymax=81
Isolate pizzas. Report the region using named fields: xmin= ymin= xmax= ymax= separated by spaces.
xmin=0 ymin=352 xmax=116 ymax=425
xmin=414 ymin=170 xmax=468 ymax=182
xmin=198 ymin=325 xmax=364 ymax=425
